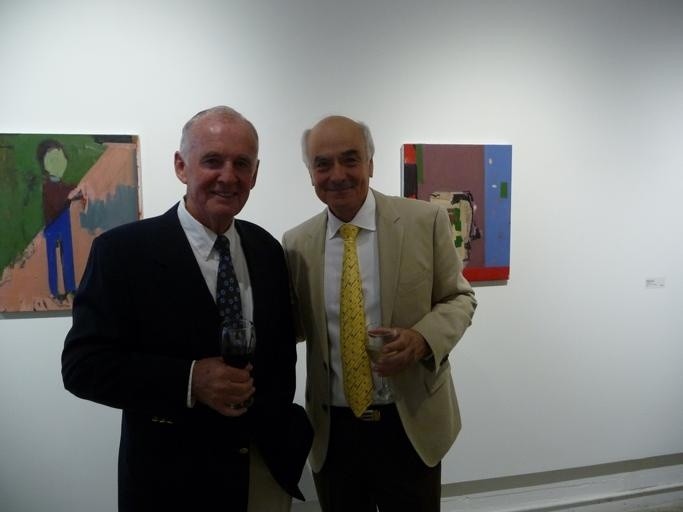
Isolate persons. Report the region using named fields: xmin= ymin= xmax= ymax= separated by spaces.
xmin=278 ymin=116 xmax=478 ymax=511
xmin=61 ymin=106 xmax=298 ymax=509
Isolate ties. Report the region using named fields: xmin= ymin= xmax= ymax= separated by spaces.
xmin=338 ymin=223 xmax=374 ymax=418
xmin=214 ymin=233 xmax=255 ymax=411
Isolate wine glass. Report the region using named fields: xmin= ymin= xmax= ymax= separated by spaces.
xmin=219 ymin=319 xmax=257 ymax=410
xmin=364 ymin=321 xmax=398 ymax=405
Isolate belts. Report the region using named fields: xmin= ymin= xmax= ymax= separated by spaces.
xmin=325 ymin=404 xmax=399 ymax=424
